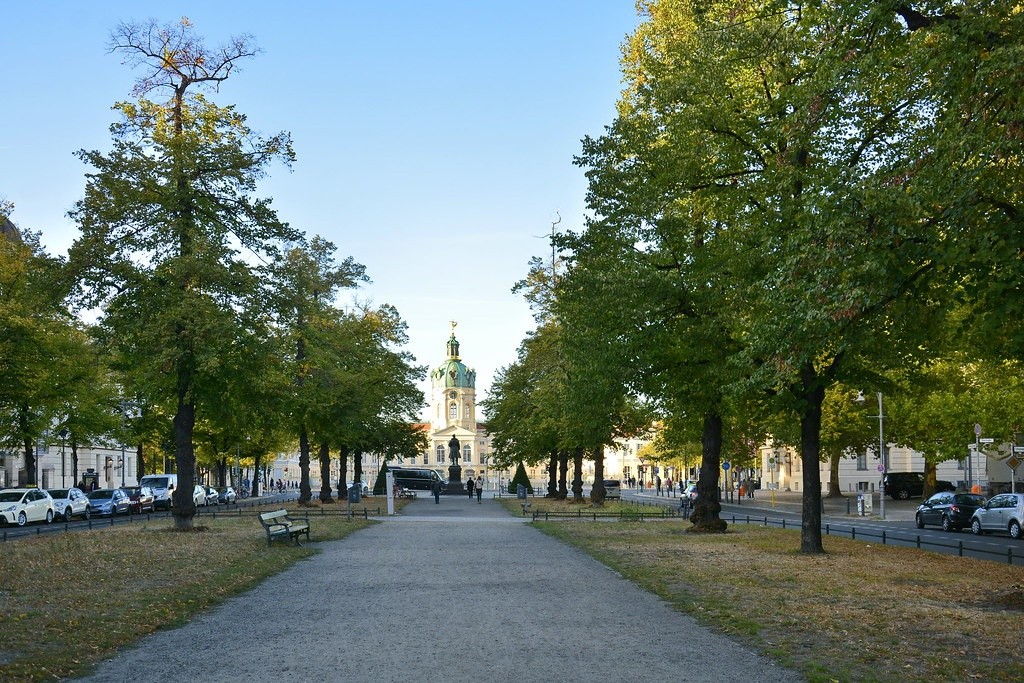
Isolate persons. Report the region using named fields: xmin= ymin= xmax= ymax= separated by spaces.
xmin=448 ymin=434 xmax=460 ymax=466
xmin=433 ymin=478 xmax=442 ymax=503
xmin=78 ymin=478 xmax=98 ymax=494
xmin=741 ymin=477 xmax=755 ymax=501
xmin=501 ymin=478 xmax=511 ymax=494
xmin=243 ymin=476 xmax=301 ymax=494
xmin=467 ymin=476 xmax=483 ymax=504
xmin=611 ymin=475 xmax=685 ymax=494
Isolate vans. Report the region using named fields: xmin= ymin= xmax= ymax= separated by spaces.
xmin=392 ymin=469 xmax=449 ymax=490
xmin=139 ymin=473 xmax=178 ymax=512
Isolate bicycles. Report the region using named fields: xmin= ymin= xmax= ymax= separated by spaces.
xmin=271 ymin=485 xmax=287 ymax=496
xmin=236 ymin=489 xmax=249 ymax=500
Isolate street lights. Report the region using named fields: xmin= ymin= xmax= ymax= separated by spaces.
xmin=58 ymin=430 xmax=68 ymax=488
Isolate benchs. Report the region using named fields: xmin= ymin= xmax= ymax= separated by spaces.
xmin=258 ymin=509 xmax=312 ymax=547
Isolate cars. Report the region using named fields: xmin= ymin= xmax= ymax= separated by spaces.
xmin=970 ymin=493 xmax=1024 ymax=540
xmin=204 ymin=487 xmax=220 ymax=506
xmin=216 ymin=487 xmax=237 ymax=505
xmin=915 ymin=491 xmax=986 ymax=533
xmin=346 ymin=480 xmax=368 ymax=491
xmin=192 ymin=484 xmax=207 ymax=508
xmin=0 ymin=488 xmax=56 ymax=527
xmin=679 ymin=484 xmax=698 ymax=509
xmin=45 ymin=487 xmax=92 ymax=523
xmin=87 ymin=488 xmax=133 ymax=518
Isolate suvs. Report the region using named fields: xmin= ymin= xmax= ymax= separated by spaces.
xmin=879 ymin=472 xmax=956 ymax=499
xmin=603 ymin=480 xmax=621 ymax=500
xmin=119 ymin=486 xmax=155 ymax=515
